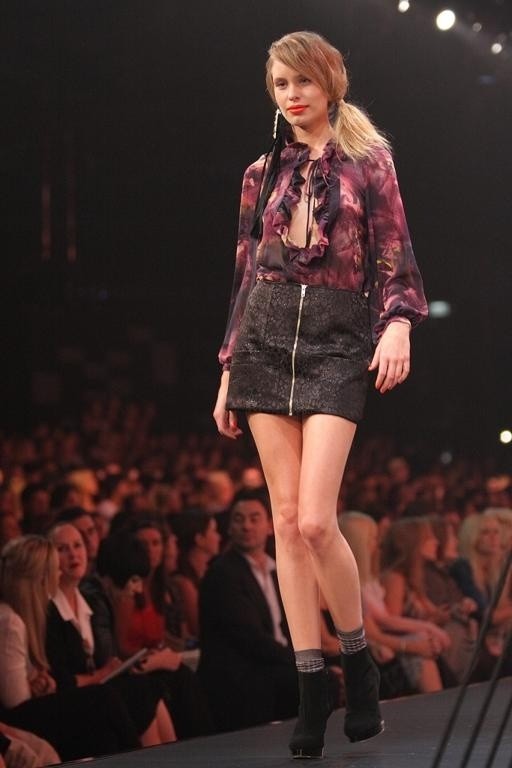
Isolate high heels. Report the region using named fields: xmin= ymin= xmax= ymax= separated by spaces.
xmin=288 ymin=665 xmax=340 ymax=759
xmin=337 ymin=644 xmax=383 ymax=742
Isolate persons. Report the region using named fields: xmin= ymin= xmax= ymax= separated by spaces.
xmin=0 ymin=722 xmax=61 ymax=768
xmin=214 ymin=29 xmax=429 ymax=759
xmin=197 ymin=488 xmax=347 ymax=732
xmin=340 ymin=510 xmax=452 ymax=694
xmin=44 ymin=520 xmax=177 ymax=748
xmin=78 ymin=529 xmax=191 ymax=746
xmin=422 ymin=516 xmax=498 ymax=680
xmin=151 ymin=521 xmax=194 ymax=646
xmin=162 ymin=509 xmax=220 ymax=641
xmin=88 ymin=400 xmax=261 ymax=538
xmin=382 ymin=516 xmax=470 ymax=694
xmin=451 ymin=514 xmax=512 ymax=677
xmin=121 ymin=511 xmax=201 ymax=740
xmin=480 ymin=508 xmax=512 ymax=592
xmin=0 ymin=402 xmax=100 ymax=572
xmin=341 ymin=433 xmax=512 ymax=542
xmin=0 ymin=536 xmax=141 ymax=761
